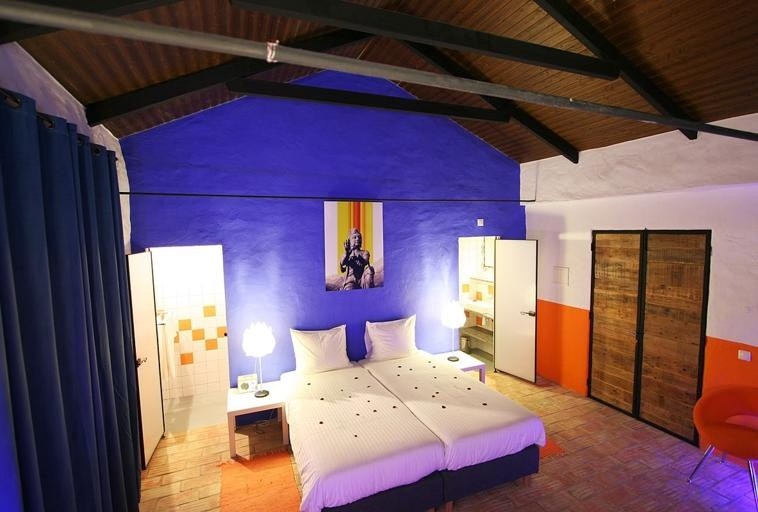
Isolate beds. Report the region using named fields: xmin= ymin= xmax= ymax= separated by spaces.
xmin=357 ymin=351 xmax=545 ymax=511
xmin=279 ymin=361 xmax=445 ymax=511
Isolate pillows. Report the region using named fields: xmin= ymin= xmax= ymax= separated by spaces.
xmin=288 ymin=313 xmax=417 ymax=375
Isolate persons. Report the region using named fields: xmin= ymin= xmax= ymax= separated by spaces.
xmin=340 ymin=228 xmax=375 ymax=291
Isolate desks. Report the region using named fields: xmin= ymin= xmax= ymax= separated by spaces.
xmin=227 ymin=379 xmax=290 ymax=457
xmin=432 ymin=349 xmax=486 ymax=384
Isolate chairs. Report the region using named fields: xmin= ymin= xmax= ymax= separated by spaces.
xmin=685 ymin=385 xmax=758 ymax=511
